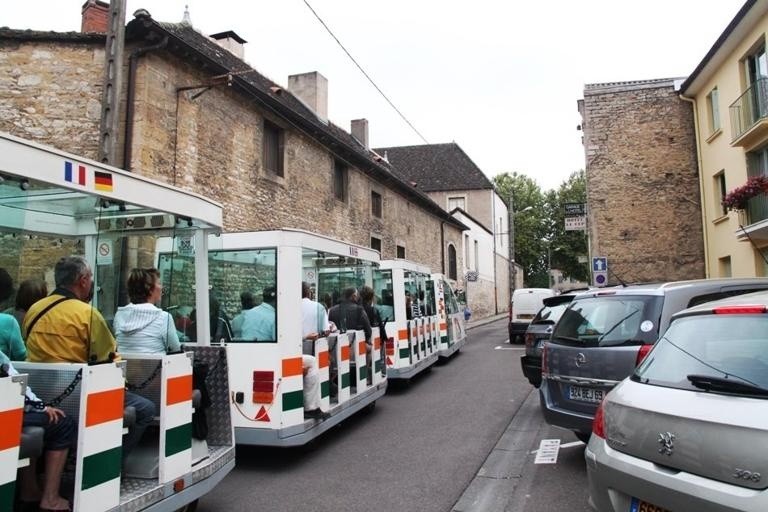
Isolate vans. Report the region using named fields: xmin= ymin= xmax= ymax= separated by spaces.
xmin=506 ymin=286 xmax=559 ymax=346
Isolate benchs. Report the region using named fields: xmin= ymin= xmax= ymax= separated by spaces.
xmin=19 ymin=424 xmax=45 ymax=459
xmin=122 ymin=404 xmax=137 ymax=429
xmin=192 ymin=389 xmax=210 ymax=466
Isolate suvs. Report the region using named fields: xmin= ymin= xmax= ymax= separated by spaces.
xmin=582 ymin=287 xmax=768 ymax=511
xmin=535 ymin=274 xmax=767 ymax=439
xmin=520 ymin=285 xmax=595 ymax=387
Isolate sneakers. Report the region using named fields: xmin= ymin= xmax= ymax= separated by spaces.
xmin=305 ymin=409 xmax=331 ymax=420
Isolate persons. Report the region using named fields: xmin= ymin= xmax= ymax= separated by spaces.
xmin=301 ymin=281 xmax=388 ymax=403
xmin=170 ymin=286 xmax=331 ymax=419
xmin=373 ymin=287 xmax=434 ymax=364
xmin=1 ymin=256 xmax=213 ymax=510
